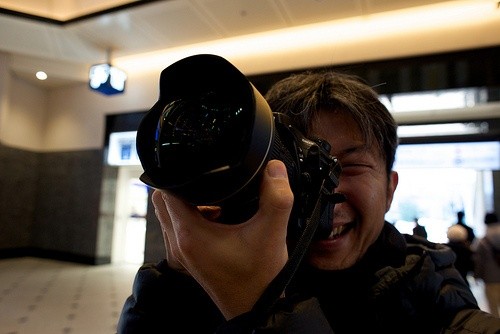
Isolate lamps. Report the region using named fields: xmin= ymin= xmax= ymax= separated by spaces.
xmin=89 ymin=47 xmax=127 ymax=96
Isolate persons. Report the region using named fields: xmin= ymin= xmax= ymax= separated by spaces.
xmin=115 ymin=71 xmax=500 ymax=334
xmin=413 ymin=212 xmax=500 ymax=320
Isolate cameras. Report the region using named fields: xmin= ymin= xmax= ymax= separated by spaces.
xmin=136 ymin=54 xmax=341 ymax=244
